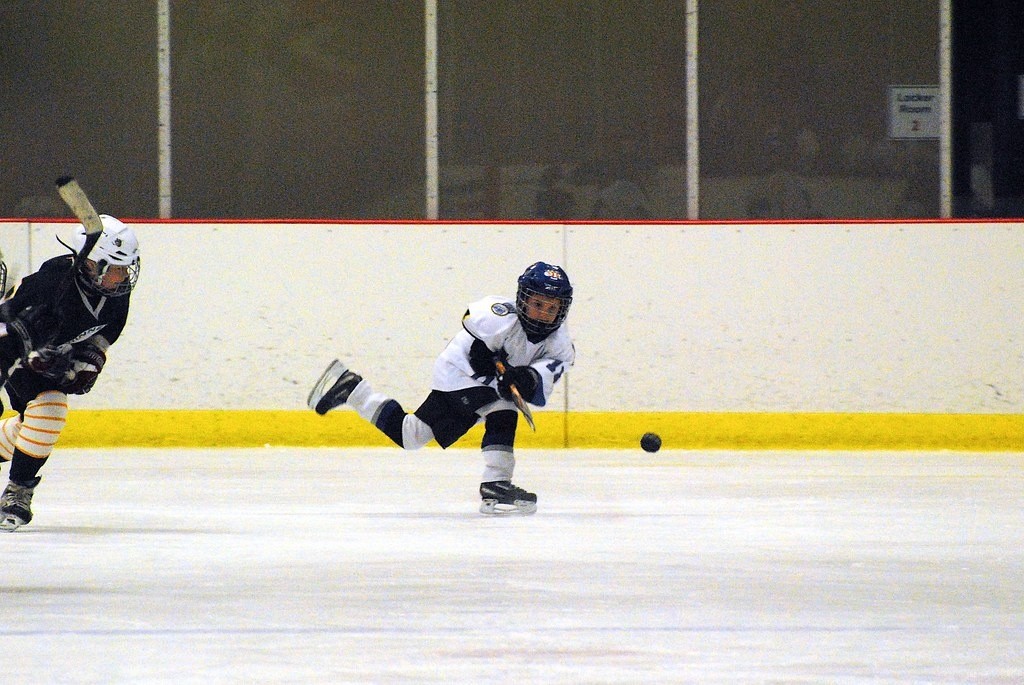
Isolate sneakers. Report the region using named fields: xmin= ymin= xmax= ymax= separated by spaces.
xmin=0 ymin=480 xmax=34 ymax=531
xmin=306 ymin=358 xmax=363 ymax=415
xmin=480 ymin=481 xmax=538 ymax=517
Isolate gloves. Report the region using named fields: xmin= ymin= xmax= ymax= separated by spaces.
xmin=497 ymin=366 xmax=538 ymax=402
xmin=61 ymin=346 xmax=106 ymax=394
xmin=469 ymin=339 xmax=498 ymax=375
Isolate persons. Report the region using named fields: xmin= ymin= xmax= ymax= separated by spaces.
xmin=0 ymin=215 xmax=140 ymax=533
xmin=308 ymin=263 xmax=575 ymax=516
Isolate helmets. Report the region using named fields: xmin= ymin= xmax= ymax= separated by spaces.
xmin=73 ymin=214 xmax=141 ymax=297
xmin=516 ymin=261 xmax=573 ymax=343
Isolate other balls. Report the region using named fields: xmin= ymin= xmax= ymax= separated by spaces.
xmin=639 ymin=432 xmax=662 ymax=454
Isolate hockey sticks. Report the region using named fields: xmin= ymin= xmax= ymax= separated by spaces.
xmin=492 ymin=353 xmax=538 ymax=433
xmin=31 ymin=175 xmax=103 ymax=351
